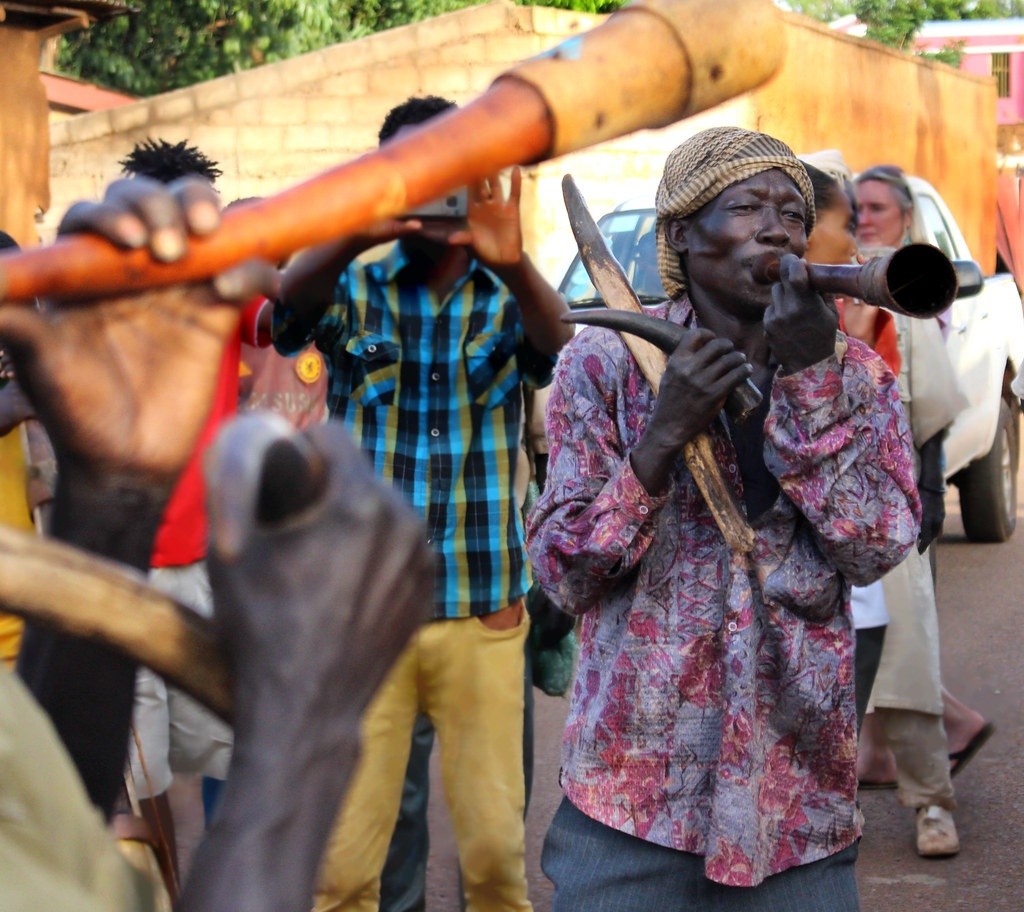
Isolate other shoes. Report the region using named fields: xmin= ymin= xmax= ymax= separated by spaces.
xmin=915 ymin=805 xmax=960 ymax=857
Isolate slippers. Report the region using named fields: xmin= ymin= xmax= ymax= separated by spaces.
xmin=857 ymin=780 xmax=899 ymax=791
xmin=949 ymin=720 xmax=997 ymax=780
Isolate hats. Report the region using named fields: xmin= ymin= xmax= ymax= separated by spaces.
xmin=654 ymin=126 xmax=817 ymax=302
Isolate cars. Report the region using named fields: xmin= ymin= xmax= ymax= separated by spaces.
xmin=555 ymin=198 xmax=670 ymax=336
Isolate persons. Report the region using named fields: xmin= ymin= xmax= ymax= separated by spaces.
xmin=523 ymin=127 xmax=923 ymax=912
xmin=0 ymin=94 xmax=573 ymax=912
xmin=800 ymin=160 xmax=994 ymax=856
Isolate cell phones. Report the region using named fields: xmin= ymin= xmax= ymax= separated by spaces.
xmin=399 ymin=184 xmax=468 ymax=220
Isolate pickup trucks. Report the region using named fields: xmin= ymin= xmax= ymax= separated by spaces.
xmin=851 ymin=171 xmax=1024 ymax=548
